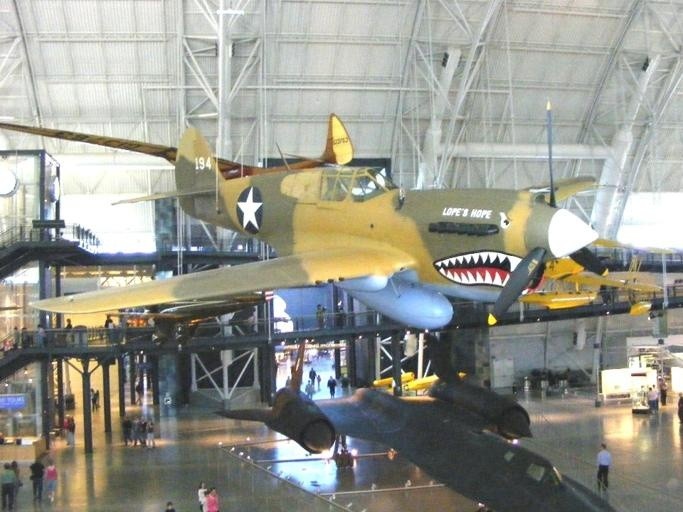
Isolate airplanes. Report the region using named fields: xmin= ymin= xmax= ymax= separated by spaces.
xmin=210 ymin=334 xmax=620 ymax=512
xmin=0 ymin=110 xmax=673 ymax=332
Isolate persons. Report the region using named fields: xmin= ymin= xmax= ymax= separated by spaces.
xmin=0 ymin=459 xmax=58 ymax=512
xmin=599 ymin=284 xmax=619 ymax=303
xmin=54 ymin=381 xmax=102 ymax=448
xmin=285 ymin=376 xmax=291 ymax=390
xmin=316 ymin=302 xmax=326 ymax=327
xmin=122 ymin=415 xmax=155 ymax=448
xmin=597 ymin=443 xmax=612 ymax=493
xmin=105 ymin=316 xmax=114 ymax=329
xmin=135 ymin=382 xmax=144 ymax=405
xmin=165 ymin=501 xmax=176 ymax=512
xmin=197 ymin=481 xmax=221 ymax=512
xmin=0 ymin=317 xmax=73 ymax=356
xmin=305 ymin=368 xmax=349 ymax=400
xmin=677 ymin=392 xmax=683 ymax=421
xmin=641 ymin=377 xmax=667 ymax=414
